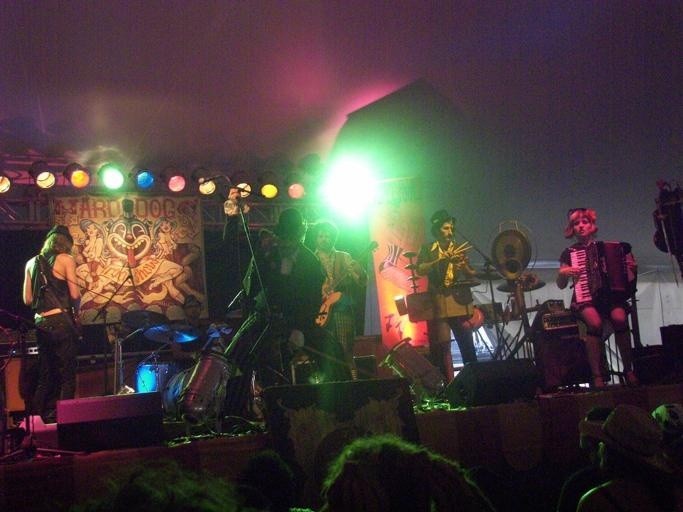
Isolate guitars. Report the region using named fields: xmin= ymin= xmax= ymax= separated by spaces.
xmin=315 ymin=241 xmax=379 ymax=328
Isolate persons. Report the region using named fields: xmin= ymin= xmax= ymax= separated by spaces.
xmin=416 ymin=209 xmax=477 ymax=386
xmin=650 ymin=404 xmax=680 ymax=474
xmin=313 ymin=220 xmax=368 ymax=367
xmin=223 ymin=207 xmax=352 ymax=432
xmin=114 ymin=460 xmax=246 ymax=509
xmin=556 ymin=207 xmax=640 ymax=389
xmin=558 ymin=408 xmax=614 ymax=508
xmin=321 ymin=431 xmax=493 ymax=509
xmin=170 ymin=294 xmax=213 ymax=373
xmin=22 ymin=223 xmax=81 ymax=424
xmin=576 ymin=404 xmax=680 ymax=509
xmin=237 ymin=449 xmax=301 ymax=509
xmin=654 ymin=178 xmax=683 ymax=279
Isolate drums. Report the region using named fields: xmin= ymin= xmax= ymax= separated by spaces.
xmin=473 ymin=303 xmax=504 ymax=323
xmin=406 ymin=292 xmax=436 ymax=323
xmin=176 ymin=350 xmax=230 ymax=425
xmin=163 ymin=363 xmax=232 ymax=429
xmin=434 ymin=290 xmax=473 ymax=318
xmin=135 ymin=362 xmax=181 ymax=393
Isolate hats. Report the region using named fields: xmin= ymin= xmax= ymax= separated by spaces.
xmin=46 ymin=224 xmax=73 ymax=245
xmin=430 ymin=209 xmax=456 ymax=236
xmin=273 ymin=208 xmax=306 ymax=237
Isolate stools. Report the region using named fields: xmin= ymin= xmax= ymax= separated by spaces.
xmin=57 ymin=391 xmax=163 ymax=451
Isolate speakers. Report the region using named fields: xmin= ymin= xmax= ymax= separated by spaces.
xmin=444 ymin=359 xmax=535 ymax=404
xmin=263 ymin=380 xmax=421 ymax=445
xmin=635 ymin=345 xmax=683 ymax=384
xmin=659 ymin=324 xmax=683 ymax=345
xmin=56 ymin=391 xmax=164 ymax=451
xmin=353 ymin=334 xmax=393 ymax=378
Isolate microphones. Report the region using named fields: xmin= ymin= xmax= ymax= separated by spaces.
xmin=123 ymin=328 xmax=145 ymax=342
xmin=452 ymin=228 xmax=457 ymax=244
xmin=198 ymin=175 xmax=223 ymax=183
xmin=0 ymin=309 xmax=36 ymax=329
xmin=126 ymin=264 xmax=135 ymax=289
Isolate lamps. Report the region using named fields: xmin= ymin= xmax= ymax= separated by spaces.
xmin=0 ymin=162 xmax=307 ymax=201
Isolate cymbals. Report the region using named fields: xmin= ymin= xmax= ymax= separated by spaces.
xmin=496 ymin=279 xmax=546 ymax=292
xmin=143 ymin=323 xmax=199 ymax=344
xmin=122 ymin=310 xmax=168 ymax=332
xmin=226 ymin=309 xmax=242 ymax=319
xmin=450 ymin=280 xmax=480 ymax=287
xmin=499 ymin=258 xmax=522 ymax=280
xmin=478 ymin=274 xmax=502 ymax=281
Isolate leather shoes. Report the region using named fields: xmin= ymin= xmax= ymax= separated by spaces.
xmin=591 ymin=374 xmax=607 ymax=392
xmin=622 ymin=369 xmax=640 ymax=387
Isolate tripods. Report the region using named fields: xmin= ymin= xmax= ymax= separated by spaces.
xmin=0 ymin=340 xmax=86 ymax=460
